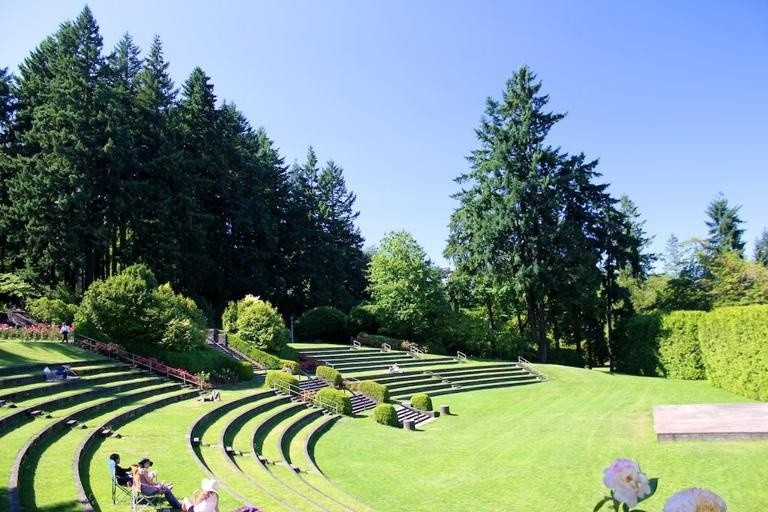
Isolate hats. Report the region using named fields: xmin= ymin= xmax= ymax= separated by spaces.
xmin=201 ymin=477 xmax=219 ymax=494
xmin=138 ymin=458 xmax=153 ymax=466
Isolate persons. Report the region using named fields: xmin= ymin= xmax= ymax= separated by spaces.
xmin=109 ymin=453 xmax=134 ymax=486
xmin=137 ymin=457 xmax=182 ymax=509
xmin=59 ymin=321 xmax=69 ymax=344
xmin=181 ymin=478 xmax=221 ymax=511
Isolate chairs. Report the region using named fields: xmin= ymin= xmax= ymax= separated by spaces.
xmin=108 ymin=459 xmax=165 ymax=512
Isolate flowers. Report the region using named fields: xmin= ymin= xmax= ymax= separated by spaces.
xmin=593 ymin=458 xmax=727 ymax=512
